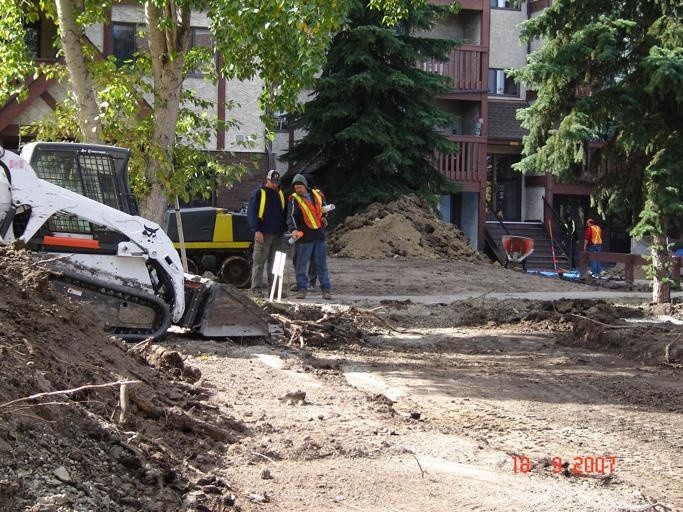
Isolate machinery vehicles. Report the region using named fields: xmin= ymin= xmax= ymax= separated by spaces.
xmin=0 ymin=144 xmax=284 ymax=350
xmin=164 ymin=201 xmax=266 ymax=287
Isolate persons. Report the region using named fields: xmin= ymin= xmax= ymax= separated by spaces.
xmin=245 ymin=169 xmax=289 ymax=298
xmin=582 ymin=218 xmax=604 ymax=276
xmin=560 ymin=207 xmax=578 ymax=266
xmin=286 ymin=173 xmax=332 ymax=300
xmin=284 ymin=174 xmax=327 ymax=292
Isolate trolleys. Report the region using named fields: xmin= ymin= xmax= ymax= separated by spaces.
xmin=501 ymin=234 xmax=536 ymax=273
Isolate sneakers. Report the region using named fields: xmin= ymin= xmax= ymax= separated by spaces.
xmin=275 ymin=290 xmax=287 ymax=299
xmin=254 ymin=291 xmax=264 ymax=298
xmin=322 ymin=292 xmax=332 ymax=299
xmin=296 ymin=290 xmax=305 ymax=299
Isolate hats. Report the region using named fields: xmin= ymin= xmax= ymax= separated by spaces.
xmin=268 ymin=169 xmax=280 ymax=180
xmin=293 ymin=181 xmax=305 ymax=185
